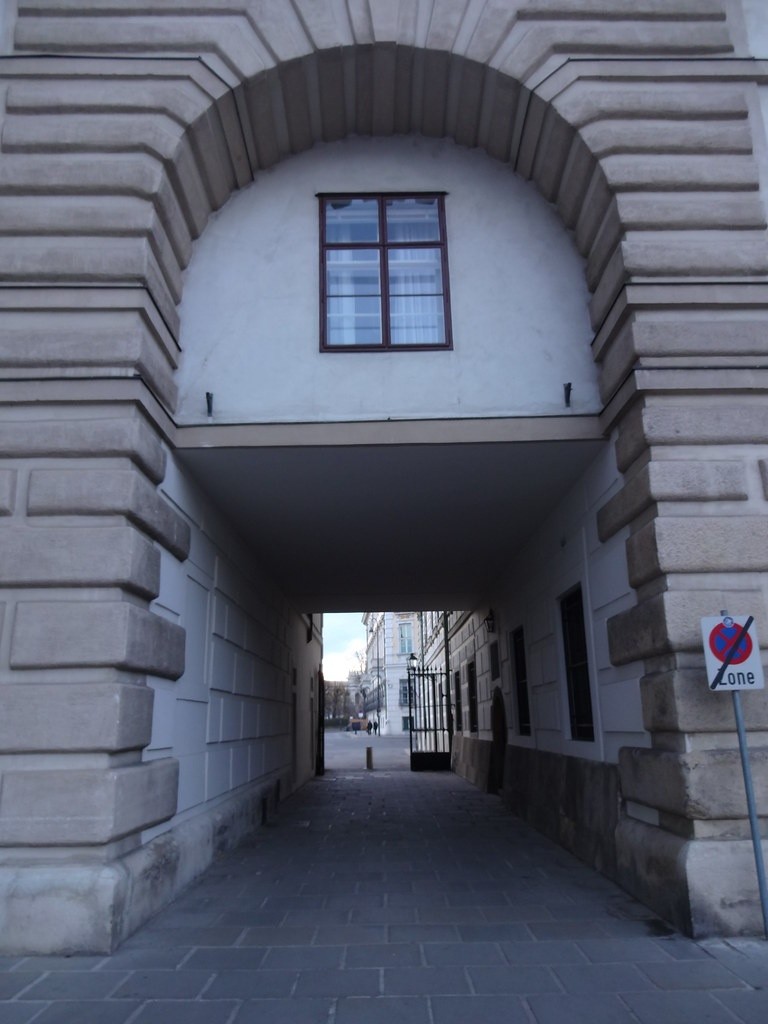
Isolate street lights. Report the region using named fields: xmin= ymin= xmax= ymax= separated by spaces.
xmin=369 ymin=617 xmax=380 ymax=736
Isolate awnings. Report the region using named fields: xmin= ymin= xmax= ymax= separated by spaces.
xmin=364 ymin=703 xmax=377 ymax=712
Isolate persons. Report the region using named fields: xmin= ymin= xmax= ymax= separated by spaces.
xmin=374 ymin=721 xmax=378 ymax=735
xmin=367 ymin=720 xmax=372 ymax=734
